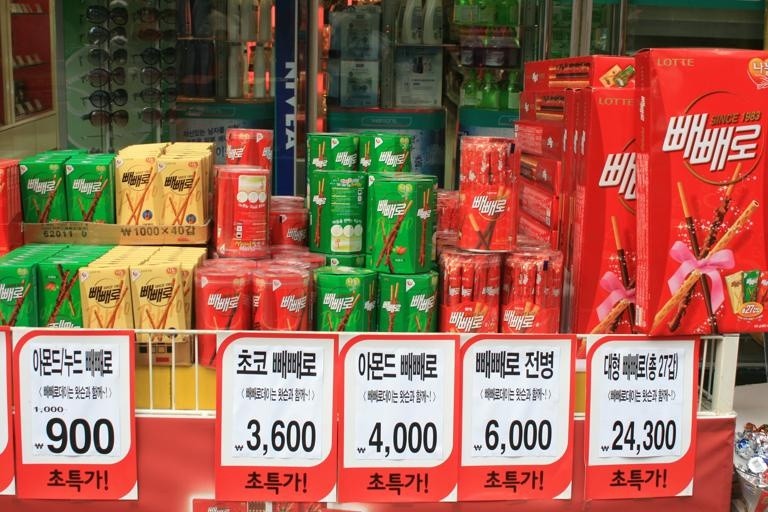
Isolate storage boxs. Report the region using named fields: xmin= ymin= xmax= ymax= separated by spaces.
xmin=519 ymin=91 xmax=568 ymax=120
xmin=524 ymin=56 xmax=636 ymax=92
xmin=520 ymin=178 xmax=561 ymax=229
xmin=520 ymin=155 xmax=562 ymax=194
xmin=562 ymin=86 xmax=635 ymax=332
xmin=519 ymin=211 xmax=560 ymax=249
xmin=636 ymin=50 xmax=768 ymax=336
xmin=514 ymin=119 xmax=561 ymax=160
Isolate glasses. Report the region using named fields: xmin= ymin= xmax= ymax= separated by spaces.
xmin=131 ymin=6 xmax=180 ymax=125
xmin=79 ymin=4 xmax=129 ymax=127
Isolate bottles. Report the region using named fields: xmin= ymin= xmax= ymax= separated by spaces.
xmin=452 ymin=0 xmax=523 ymax=112
